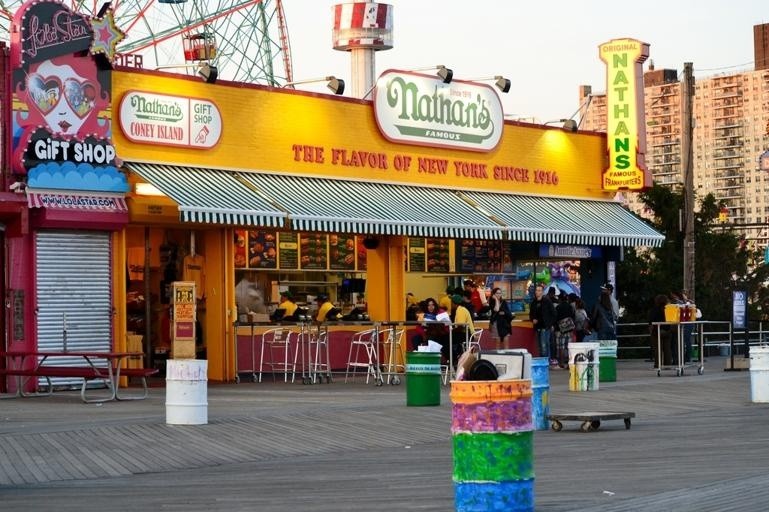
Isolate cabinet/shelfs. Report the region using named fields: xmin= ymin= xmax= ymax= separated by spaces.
xmin=234 ymin=230 xmax=367 ymax=273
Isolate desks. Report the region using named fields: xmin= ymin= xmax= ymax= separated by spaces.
xmin=0 ymin=350 xmax=159 ymax=404
xmin=652 ymin=320 xmax=708 ymax=377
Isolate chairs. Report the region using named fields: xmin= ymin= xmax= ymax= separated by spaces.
xmin=440 ymin=327 xmax=483 ymax=386
xmin=259 ymin=325 xmax=406 ymax=387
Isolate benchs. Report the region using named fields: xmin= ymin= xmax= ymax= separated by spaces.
xmin=0 ymin=364 xmax=159 ymax=379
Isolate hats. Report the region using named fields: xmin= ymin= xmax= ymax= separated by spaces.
xmin=558 ymin=293 xmax=568 ymax=300
xmin=280 ymin=291 xmax=292 ymax=298
xmin=600 ymin=283 xmax=613 ymax=290
xmin=448 ymin=286 xmax=455 ymax=291
xmin=314 ymin=295 xmax=328 ymax=301
xmin=449 ymin=295 xmax=463 ymax=304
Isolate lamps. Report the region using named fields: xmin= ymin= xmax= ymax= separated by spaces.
xmin=539 ymin=117 xmax=577 ymax=134
xmin=280 ymin=74 xmax=346 ymax=96
xmin=155 ymin=62 xmax=219 ymax=85
xmin=466 ymin=74 xmax=513 ymax=94
xmin=134 ymin=182 xmax=167 ymax=196
xmin=361 ymin=65 xmax=455 ymax=104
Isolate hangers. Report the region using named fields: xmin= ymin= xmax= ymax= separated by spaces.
xmin=188 ymin=246 xmax=197 ymax=258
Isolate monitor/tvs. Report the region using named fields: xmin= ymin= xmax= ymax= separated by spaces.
xmin=350 ymin=304 xmax=369 ymax=316
xmin=325 ymin=307 xmax=343 ymax=320
xmin=271 ymin=308 xmax=285 ymax=318
xmin=343 ymin=278 xmax=366 ymax=293
xmin=293 ymin=306 xmax=309 ymax=314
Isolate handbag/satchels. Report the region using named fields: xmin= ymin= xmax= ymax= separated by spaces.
xmin=557 ymin=317 xmax=575 ymax=333
xmin=596 ymin=317 xmax=613 ymax=333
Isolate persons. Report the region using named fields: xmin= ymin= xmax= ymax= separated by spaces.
xmin=273 ymin=291 xmax=299 ymax=322
xmin=314 ymin=294 xmax=342 ymax=324
xmin=14 ymin=50 xmax=110 ymax=175
xmin=407 ymin=280 xmax=695 ymax=372
